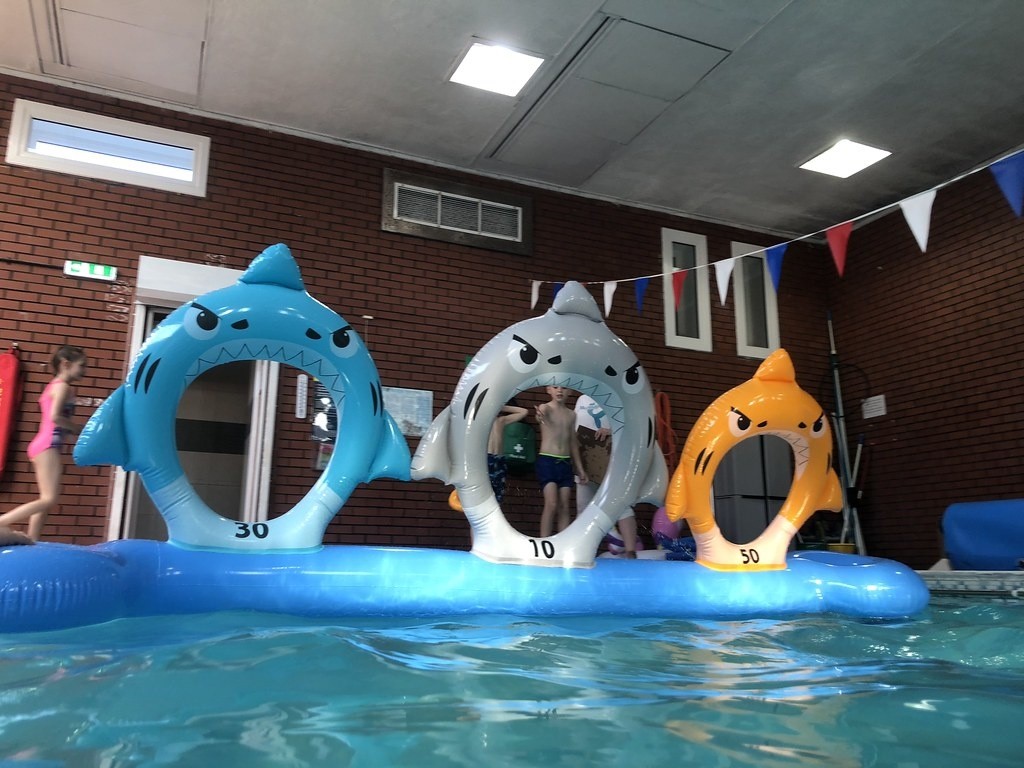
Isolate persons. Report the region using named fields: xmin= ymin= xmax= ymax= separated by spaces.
xmin=533 ymin=384 xmax=588 ymax=539
xmin=0 ymin=345 xmax=92 ymax=543
xmin=486 ymin=404 xmax=529 ymax=507
xmin=573 ymin=394 xmax=637 ymax=561
